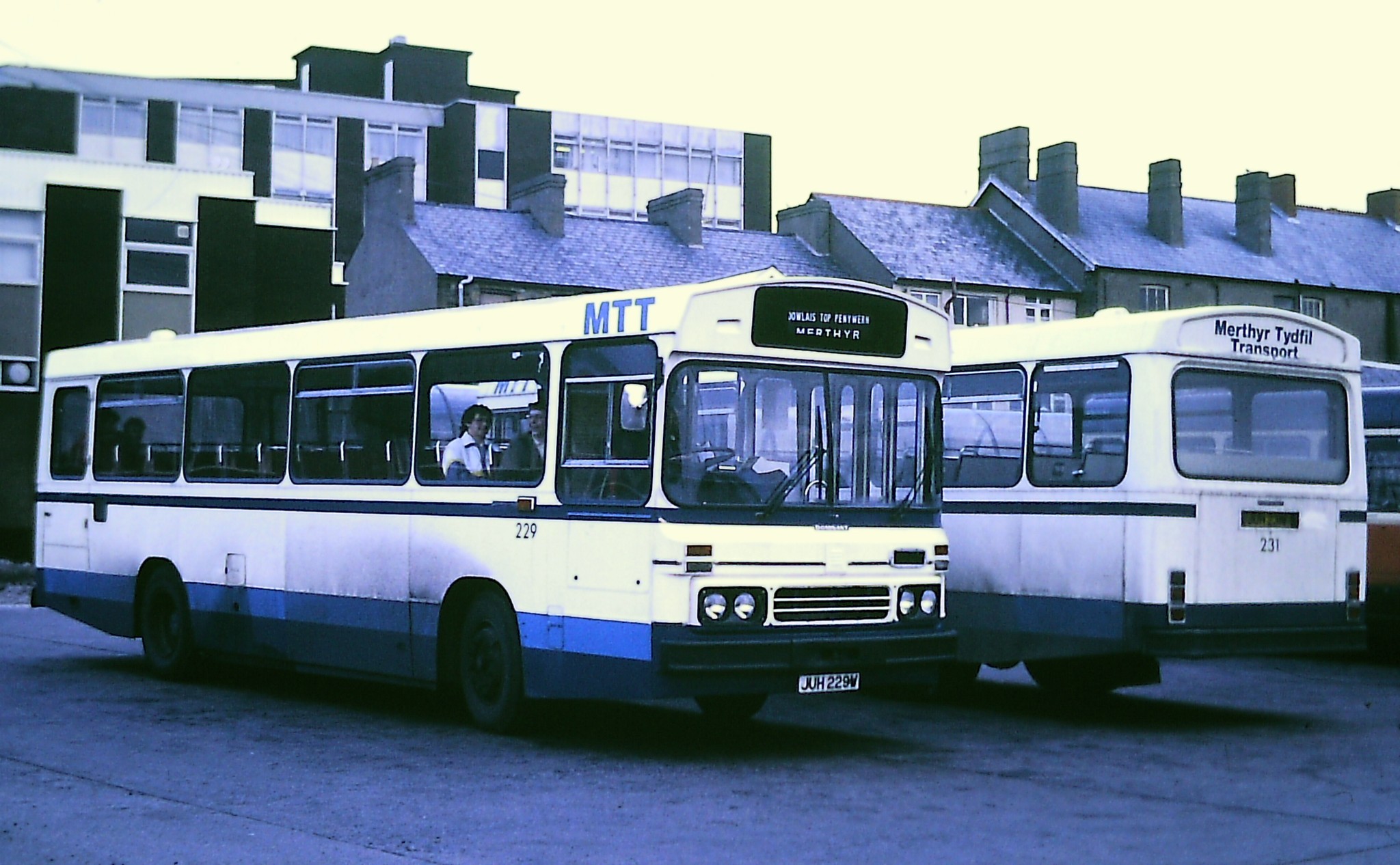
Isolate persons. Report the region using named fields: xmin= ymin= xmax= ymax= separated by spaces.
xmin=500 ymin=403 xmax=547 ymax=467
xmin=59 ymin=407 xmax=147 ymax=474
xmin=442 ymin=404 xmax=494 ymax=479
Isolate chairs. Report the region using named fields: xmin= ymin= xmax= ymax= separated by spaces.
xmin=112 ymin=438 xmax=510 ymax=481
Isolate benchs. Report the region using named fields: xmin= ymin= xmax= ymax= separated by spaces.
xmin=897 ymin=445 xmax=1345 ymax=485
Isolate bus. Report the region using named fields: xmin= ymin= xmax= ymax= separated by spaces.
xmin=29 ymin=263 xmax=1399 ymax=737
